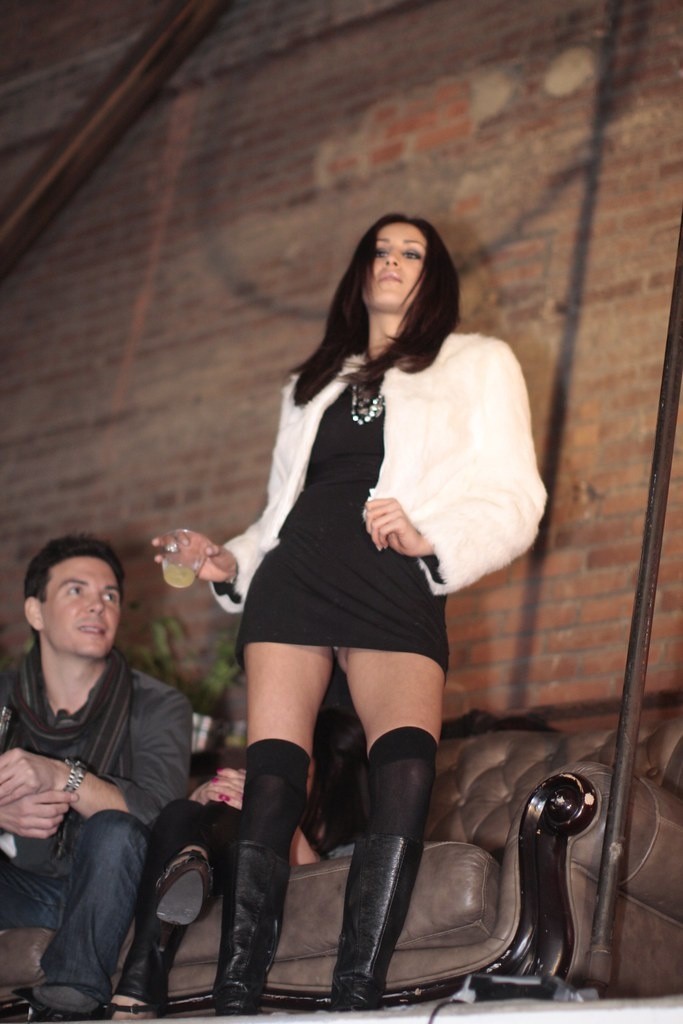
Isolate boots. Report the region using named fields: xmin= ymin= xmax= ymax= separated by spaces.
xmin=212 ymin=839 xmax=291 ymax=1016
xmin=330 ymin=834 xmax=423 ymax=1012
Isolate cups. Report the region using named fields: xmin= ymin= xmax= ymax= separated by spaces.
xmin=161 ymin=527 xmax=209 ymax=588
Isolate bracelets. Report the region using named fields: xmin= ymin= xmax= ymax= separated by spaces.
xmin=63 ymin=758 xmax=87 ymax=793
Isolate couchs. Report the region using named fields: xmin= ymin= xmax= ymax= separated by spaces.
xmin=0 ymin=730 xmax=683 ymax=1023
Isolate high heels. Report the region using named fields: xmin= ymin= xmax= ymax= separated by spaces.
xmin=153 ymin=862 xmax=210 ymax=953
xmin=103 ymin=1002 xmax=162 ymax=1020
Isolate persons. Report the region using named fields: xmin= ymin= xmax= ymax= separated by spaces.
xmin=103 ymin=702 xmax=366 ymax=1020
xmin=0 ymin=533 xmax=191 ymax=1024
xmin=152 ymin=213 xmax=547 ymax=1016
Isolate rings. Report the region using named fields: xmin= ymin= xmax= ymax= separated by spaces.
xmin=166 ymin=543 xmax=176 ymax=553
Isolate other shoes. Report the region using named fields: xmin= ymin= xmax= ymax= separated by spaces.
xmin=28 ymin=1003 xmax=103 ymax=1022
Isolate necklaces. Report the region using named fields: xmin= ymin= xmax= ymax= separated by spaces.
xmin=351 ymin=382 xmax=382 ymax=425
